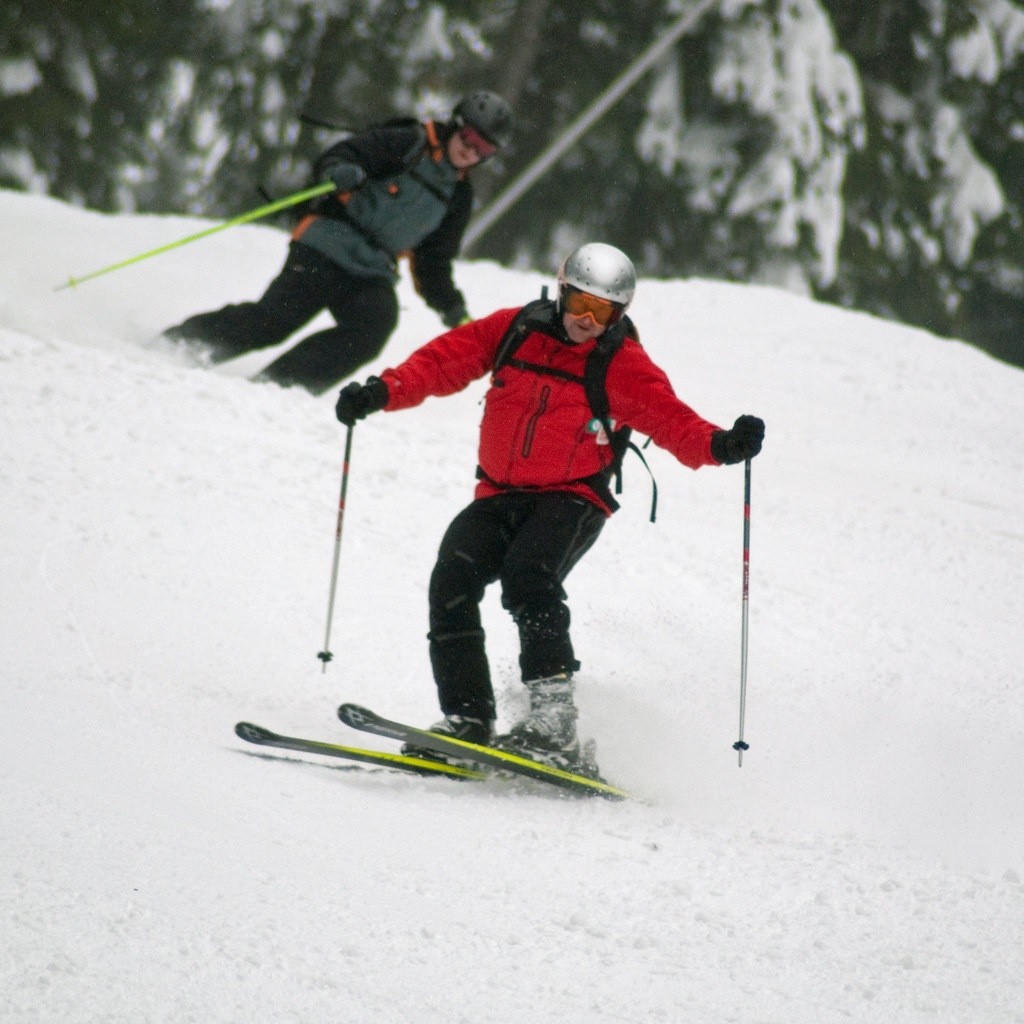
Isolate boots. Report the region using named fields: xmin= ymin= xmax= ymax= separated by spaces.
xmin=489 ymin=670 xmax=580 ymax=772
xmin=399 ymin=713 xmax=497 ymax=774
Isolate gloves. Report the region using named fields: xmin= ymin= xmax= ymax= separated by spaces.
xmin=443 ymin=305 xmax=467 ymax=329
xmin=329 ymin=162 xmax=364 ymax=193
xmin=335 ymin=376 xmax=390 ymax=427
xmin=710 ymin=414 xmax=765 ymax=465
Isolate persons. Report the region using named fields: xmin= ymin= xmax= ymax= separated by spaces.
xmin=160 ymin=89 xmax=516 ymax=399
xmin=336 ymin=240 xmax=765 ymax=775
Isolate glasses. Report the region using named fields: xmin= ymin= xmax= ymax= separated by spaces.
xmin=461 ymin=125 xmax=497 ymax=158
xmin=564 ymin=288 xmax=623 ymax=327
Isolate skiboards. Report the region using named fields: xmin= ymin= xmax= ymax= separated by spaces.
xmin=233 ymin=706 xmax=636 ymax=810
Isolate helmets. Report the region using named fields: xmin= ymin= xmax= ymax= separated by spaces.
xmin=559 ymin=242 xmax=636 ymax=319
xmin=454 ymin=89 xmax=518 ymax=153
